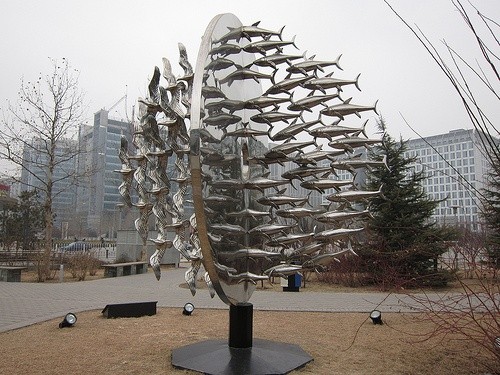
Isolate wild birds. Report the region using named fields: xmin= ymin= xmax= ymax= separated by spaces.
xmin=114 ymin=42 xmax=216 ymax=301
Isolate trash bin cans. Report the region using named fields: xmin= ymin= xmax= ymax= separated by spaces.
xmin=279 ymin=260 xmax=304 ymax=292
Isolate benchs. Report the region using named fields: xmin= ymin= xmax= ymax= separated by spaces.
xmin=0 ymin=266 xmax=29 ymax=282
xmin=101 ymin=261 xmax=149 ymax=278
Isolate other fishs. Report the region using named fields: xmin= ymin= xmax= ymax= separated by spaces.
xmin=241 ymin=20 xmax=391 ymax=285
xmin=204 ymin=26 xmax=240 ymax=286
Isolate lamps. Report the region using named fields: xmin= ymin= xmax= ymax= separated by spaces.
xmin=370 ymin=310 xmax=384 ymax=324
xmin=184 ymin=303 xmax=195 ymax=316
xmin=58 ymin=313 xmax=77 ymax=328
